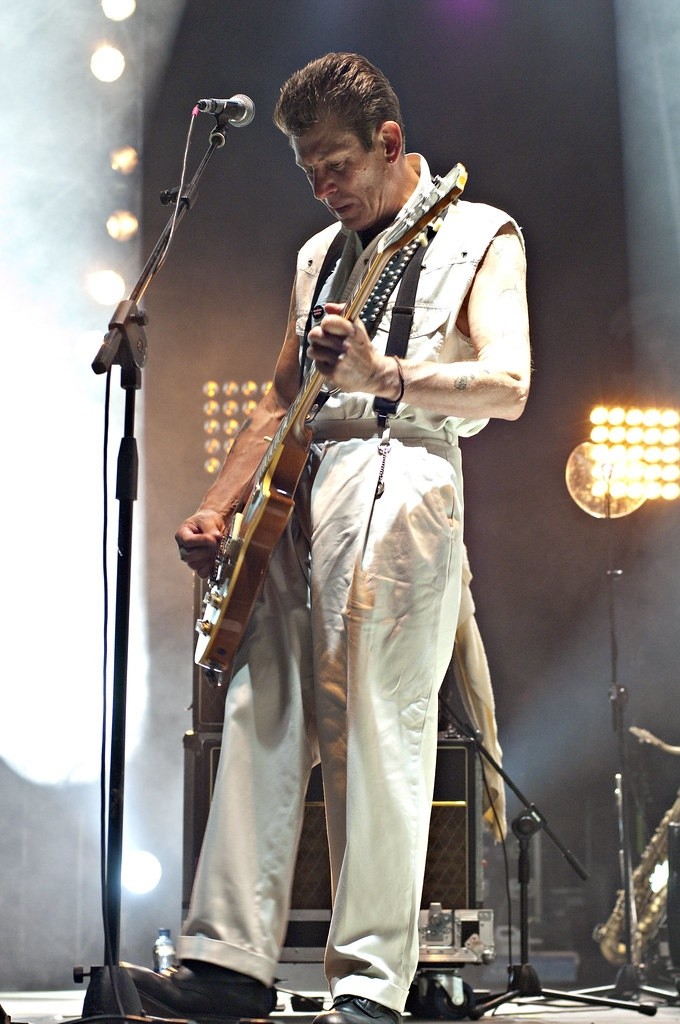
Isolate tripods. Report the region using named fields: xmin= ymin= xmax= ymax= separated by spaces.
xmin=438 ymin=494 xmax=680 ymax=1019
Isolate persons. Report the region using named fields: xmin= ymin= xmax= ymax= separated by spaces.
xmin=120 ymin=52 xmax=532 ymax=1024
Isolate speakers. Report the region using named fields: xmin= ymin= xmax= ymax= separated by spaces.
xmin=182 ymin=732 xmax=486 ymax=913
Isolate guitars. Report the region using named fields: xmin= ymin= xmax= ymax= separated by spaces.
xmin=194 ymin=160 xmax=468 ymax=674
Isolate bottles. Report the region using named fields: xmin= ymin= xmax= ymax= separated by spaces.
xmin=426 ymin=902 xmax=447 ymax=939
xmin=153 ymin=928 xmax=177 ymax=976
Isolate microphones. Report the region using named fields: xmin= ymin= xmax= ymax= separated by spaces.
xmin=197 ymin=94 xmax=255 ymax=127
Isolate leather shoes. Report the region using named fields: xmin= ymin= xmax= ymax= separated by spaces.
xmin=312 ymin=991 xmax=400 ymax=1024
xmin=115 ymin=952 xmax=277 ymax=1023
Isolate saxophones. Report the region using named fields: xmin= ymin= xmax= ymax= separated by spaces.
xmin=591 ymin=726 xmax=680 ymax=965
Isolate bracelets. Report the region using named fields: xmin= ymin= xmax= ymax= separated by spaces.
xmin=386 ymin=348 xmax=406 ymax=403
